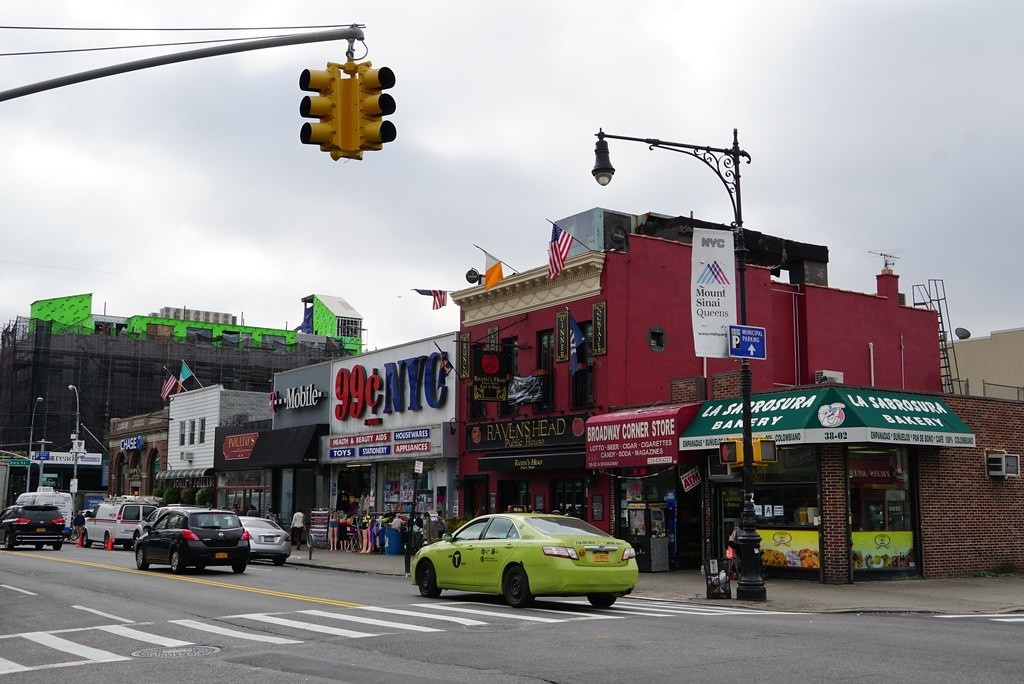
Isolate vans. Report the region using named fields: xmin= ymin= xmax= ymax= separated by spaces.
xmin=15 ymin=486 xmax=73 ymax=542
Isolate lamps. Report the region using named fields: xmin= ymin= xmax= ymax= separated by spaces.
xmin=547 ymin=405 xmax=564 ymax=423
xmin=511 ymin=409 xmax=528 ymax=425
xmin=453 ymin=470 xmax=464 ymax=492
xmin=582 ymin=401 xmax=603 ymax=419
xmin=449 ymin=418 xmax=467 ymax=436
xmin=479 ymin=414 xmax=494 ymax=431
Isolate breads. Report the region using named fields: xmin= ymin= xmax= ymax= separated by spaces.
xmin=799 ymin=549 xmax=820 ymax=569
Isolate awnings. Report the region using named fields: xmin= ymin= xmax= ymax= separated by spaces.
xmin=246 ymin=423 xmax=331 ymax=466
xmin=679 ymin=388 xmax=976 ymax=447
xmin=156 ymin=468 xmax=213 ymax=479
xmin=586 ymin=402 xmax=701 ymax=469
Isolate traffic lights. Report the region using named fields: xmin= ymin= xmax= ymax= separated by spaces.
xmin=298 ymin=65 xmax=343 ymax=152
xmin=347 ymin=64 xmax=397 ymax=153
xmin=754 ymin=438 xmax=778 ymax=463
xmin=717 ymin=440 xmax=744 ymax=463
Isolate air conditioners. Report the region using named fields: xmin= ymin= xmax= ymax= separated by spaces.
xmin=988 ymin=454 xmax=1020 ymax=477
xmin=814 ymin=369 xmax=844 ymax=384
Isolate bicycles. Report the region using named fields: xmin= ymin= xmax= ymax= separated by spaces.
xmin=71 ymin=532 xmax=78 ymax=541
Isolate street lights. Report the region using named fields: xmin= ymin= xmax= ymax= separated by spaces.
xmin=25 ymin=396 xmax=44 ymax=494
xmin=68 ymin=384 xmax=81 ymax=518
xmin=588 ymin=125 xmax=773 ymax=602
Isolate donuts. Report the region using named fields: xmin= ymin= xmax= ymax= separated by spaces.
xmin=770 ymin=552 xmax=788 ymax=566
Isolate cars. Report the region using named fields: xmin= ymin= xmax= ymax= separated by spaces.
xmin=134 ymin=508 xmax=252 ymax=575
xmin=215 ymin=515 xmax=292 ymax=566
xmin=410 ymin=512 xmax=639 ymax=609
xmin=132 ymin=506 xmax=200 ymax=551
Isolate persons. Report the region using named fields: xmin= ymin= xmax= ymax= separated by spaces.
xmin=329 ymin=519 xmax=338 ymax=550
xmin=729 ymin=518 xmax=743 ymax=575
xmin=361 ymin=520 xmax=371 ymax=553
xmin=74 ymin=511 xmax=85 ymax=538
xmin=222 ymin=503 xmax=278 ymax=524
xmin=290 ymin=508 xmax=305 ymax=550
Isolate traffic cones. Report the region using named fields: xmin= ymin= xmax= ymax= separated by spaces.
xmin=106 ymin=537 xmax=114 ymax=551
xmin=76 ymin=533 xmax=85 ymax=549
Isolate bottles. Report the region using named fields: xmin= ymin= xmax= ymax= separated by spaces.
xmin=869 ymin=502 xmax=901 ymax=512
xmin=814 ymin=514 xmax=821 ymax=525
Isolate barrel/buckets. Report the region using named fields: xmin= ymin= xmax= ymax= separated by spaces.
xmin=385 ymin=528 xmax=402 ymax=555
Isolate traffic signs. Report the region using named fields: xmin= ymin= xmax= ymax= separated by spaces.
xmin=728 ymin=324 xmax=769 ymax=360
xmin=34 ymin=450 xmax=50 ymax=461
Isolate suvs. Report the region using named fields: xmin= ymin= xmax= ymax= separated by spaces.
xmin=80 ymin=496 xmax=160 ymax=549
xmin=0 ymin=503 xmax=67 ymax=551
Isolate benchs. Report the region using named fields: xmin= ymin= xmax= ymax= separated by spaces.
xmin=204 ymin=519 xmax=228 ymax=526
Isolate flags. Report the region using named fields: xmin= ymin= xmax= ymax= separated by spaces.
xmin=485 ymin=252 xmax=503 ymax=290
xmin=177 ymin=362 xmax=192 ymax=394
xmin=416 ymin=290 xmax=447 ymax=310
xmin=549 ymin=224 xmax=574 ymax=279
xmin=160 ymin=369 xmax=176 ymax=399
